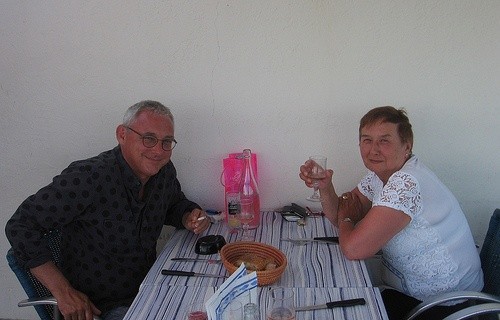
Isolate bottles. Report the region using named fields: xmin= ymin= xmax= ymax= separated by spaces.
xmin=229 ymin=300 xmax=259 ymax=320
xmin=238 ymin=149 xmax=261 ymax=230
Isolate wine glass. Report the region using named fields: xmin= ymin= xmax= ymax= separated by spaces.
xmin=305 ymin=155 xmax=328 ymax=202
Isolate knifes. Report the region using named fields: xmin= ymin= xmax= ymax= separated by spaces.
xmin=281 ymin=236 xmax=339 ymax=245
xmin=161 ymin=269 xmax=229 ymax=278
xmin=295 ymin=298 xmax=366 ymax=311
xmin=171 ymin=257 xmax=220 ymax=263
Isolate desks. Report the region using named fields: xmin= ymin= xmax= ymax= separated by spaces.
xmin=122 ymin=209 xmax=391 ymax=320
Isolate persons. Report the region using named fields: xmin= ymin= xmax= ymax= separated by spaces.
xmin=299 ymin=106 xmax=486 ymax=320
xmin=5 ymin=100 xmax=212 ymax=320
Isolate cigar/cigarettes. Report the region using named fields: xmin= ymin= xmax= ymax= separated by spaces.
xmin=191 ymin=216 xmax=207 ymax=223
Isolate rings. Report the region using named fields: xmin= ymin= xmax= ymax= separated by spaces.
xmin=342 ymin=196 xmax=349 ymax=200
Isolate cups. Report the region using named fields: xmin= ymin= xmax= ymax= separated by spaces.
xmin=265 ymin=288 xmax=297 ymax=320
xmin=182 ymin=302 xmax=212 ymax=320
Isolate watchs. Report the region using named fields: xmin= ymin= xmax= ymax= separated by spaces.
xmin=338 ymin=217 xmax=355 ymax=225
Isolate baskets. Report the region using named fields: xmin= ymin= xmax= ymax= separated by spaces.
xmin=221 ymin=241 xmax=287 ymax=286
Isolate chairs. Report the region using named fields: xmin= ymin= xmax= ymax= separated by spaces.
xmin=407 ymin=208 xmax=500 ymax=320
xmin=6 ymin=226 xmax=102 ymax=320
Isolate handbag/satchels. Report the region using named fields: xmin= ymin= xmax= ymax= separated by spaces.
xmin=220 ymin=153 xmax=260 ymax=226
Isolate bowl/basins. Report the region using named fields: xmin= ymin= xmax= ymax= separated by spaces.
xmin=219 ymin=241 xmax=287 ymax=286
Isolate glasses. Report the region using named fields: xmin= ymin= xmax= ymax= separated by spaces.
xmin=126 ymin=126 xmax=178 ymax=151
